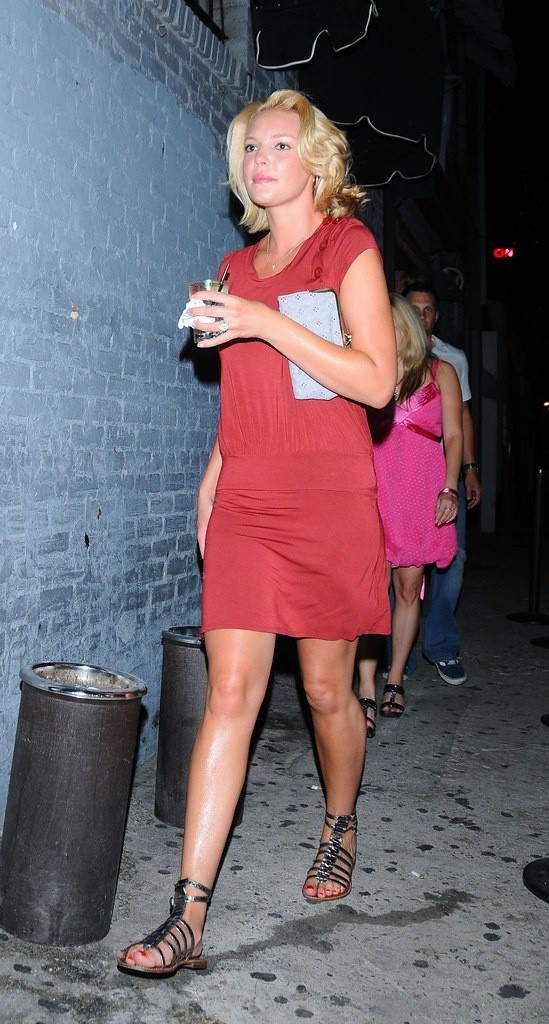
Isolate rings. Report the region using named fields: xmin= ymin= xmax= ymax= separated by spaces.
xmin=221 ymin=320 xmax=227 ymax=332
xmin=445 ymin=509 xmax=452 ymax=512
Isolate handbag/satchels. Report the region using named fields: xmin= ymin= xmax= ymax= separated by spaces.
xmin=278 ymin=287 xmax=351 ymax=401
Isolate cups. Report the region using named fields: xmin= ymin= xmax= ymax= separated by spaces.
xmin=188 ymin=279 xmax=228 ymax=344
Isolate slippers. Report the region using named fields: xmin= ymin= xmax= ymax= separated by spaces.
xmin=357 ymin=698 xmax=377 ymax=738
xmin=380 ymin=684 xmax=405 ymax=718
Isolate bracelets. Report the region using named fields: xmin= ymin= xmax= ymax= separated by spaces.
xmin=463 ymin=464 xmax=478 ymax=472
xmin=438 ymin=488 xmax=458 ymax=499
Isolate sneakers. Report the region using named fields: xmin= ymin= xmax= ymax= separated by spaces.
xmin=434 ymin=654 xmax=467 ymax=685
xmin=382 ymin=666 xmax=408 ymax=681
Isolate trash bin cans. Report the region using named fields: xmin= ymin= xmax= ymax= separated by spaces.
xmin=153 ymin=624 xmax=244 ymax=830
xmin=0 ymin=660 xmax=148 ymax=945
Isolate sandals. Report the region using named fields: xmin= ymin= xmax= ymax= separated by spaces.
xmin=117 ymin=878 xmax=211 ymax=973
xmin=301 ymin=810 xmax=358 ymax=900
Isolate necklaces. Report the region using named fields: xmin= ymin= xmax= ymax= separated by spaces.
xmin=268 ymin=220 xmax=323 ymax=270
xmin=394 ymin=387 xmax=398 ymax=396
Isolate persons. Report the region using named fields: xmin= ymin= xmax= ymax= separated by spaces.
xmin=356 ymin=294 xmax=463 ymax=736
xmin=118 ymin=90 xmax=396 ymax=974
xmin=386 ymin=282 xmax=479 ymax=686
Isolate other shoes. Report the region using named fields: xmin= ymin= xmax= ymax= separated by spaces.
xmin=539 ymin=713 xmax=549 ymax=727
